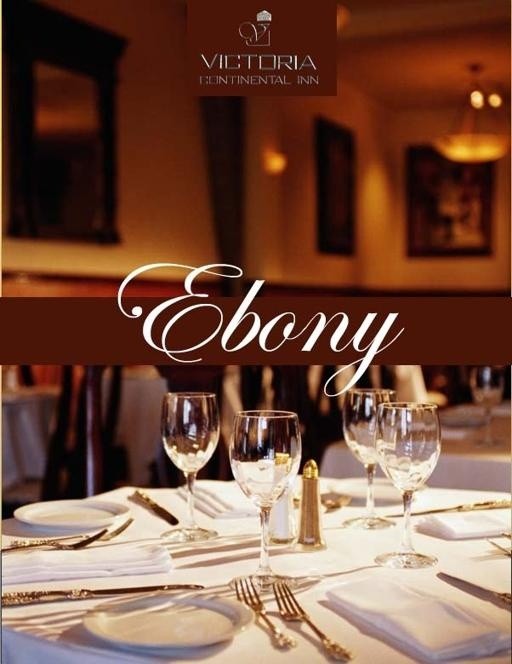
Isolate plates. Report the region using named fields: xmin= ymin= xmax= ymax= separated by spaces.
xmin=326 ymin=477 xmax=430 ymax=500
xmin=80 ymin=587 xmax=256 ymax=649
xmin=13 ymin=499 xmax=129 ymax=531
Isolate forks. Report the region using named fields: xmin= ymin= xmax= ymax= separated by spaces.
xmin=1 ymin=529 xmax=108 ymax=554
xmin=486 ymin=538 xmax=512 ymax=556
xmin=12 ymin=518 xmax=134 ymax=547
xmin=271 ymin=583 xmax=354 ymax=662
xmin=234 ymin=578 xmax=298 ymax=649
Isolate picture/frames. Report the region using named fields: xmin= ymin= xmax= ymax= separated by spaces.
xmin=404 ymin=143 xmax=494 ymax=257
xmin=316 ymin=117 xmax=356 ymax=255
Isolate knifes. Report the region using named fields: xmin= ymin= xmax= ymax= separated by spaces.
xmin=1 ymin=585 xmax=204 ymax=606
xmin=385 ymin=499 xmax=511 ymax=518
xmin=135 ymin=490 xmax=179 ymax=526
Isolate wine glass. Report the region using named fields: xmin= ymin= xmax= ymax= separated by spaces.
xmin=228 ymin=410 xmax=302 ymax=595
xmin=373 ymin=400 xmax=442 ymax=570
xmin=159 ymin=391 xmax=219 ymax=542
xmin=469 ymin=364 xmax=504 ymax=448
xmin=341 ymin=388 xmax=398 ymax=531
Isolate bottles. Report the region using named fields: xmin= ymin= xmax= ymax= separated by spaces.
xmin=270 ymin=460 xmax=322 ymax=548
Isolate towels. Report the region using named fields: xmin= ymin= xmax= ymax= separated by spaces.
xmin=417 ymin=509 xmax=512 ymax=539
xmin=326 ymin=575 xmax=512 ymax=664
xmin=178 ymin=480 xmax=260 ymax=519
xmin=0 ymin=545 xmax=174 ymax=586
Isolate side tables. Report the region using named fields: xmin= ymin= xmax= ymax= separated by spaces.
xmin=3 ymin=369 xmax=512 ymax=502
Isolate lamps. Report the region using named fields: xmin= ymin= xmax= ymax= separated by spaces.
xmin=431 ymin=63 xmax=511 ymax=163
